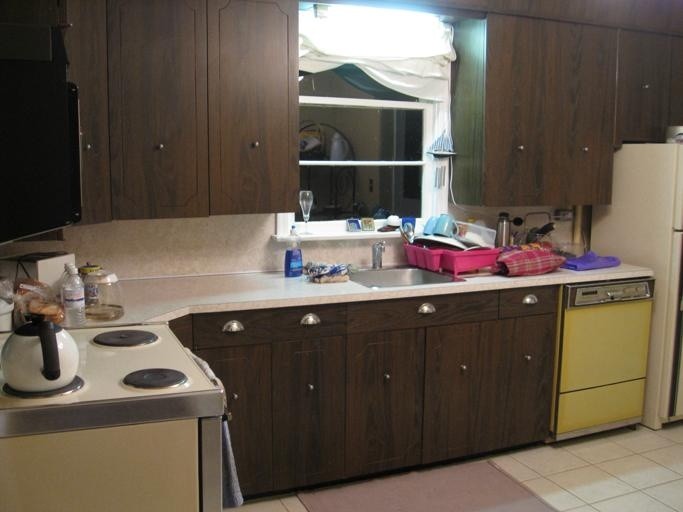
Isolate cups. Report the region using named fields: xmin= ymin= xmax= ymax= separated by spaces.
xmin=433 ymin=213 xmax=458 ymax=238
xmin=421 ymin=216 xmax=438 ymax=235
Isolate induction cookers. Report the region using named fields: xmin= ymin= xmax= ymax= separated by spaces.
xmin=0 ymin=321 xmax=225 ymax=437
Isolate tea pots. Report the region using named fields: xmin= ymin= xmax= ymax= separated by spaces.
xmin=1 ymin=314 xmax=80 ymax=393
xmin=328 ymin=132 xmax=350 ymax=162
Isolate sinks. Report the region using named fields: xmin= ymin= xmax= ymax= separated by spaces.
xmin=347 ymin=265 xmax=466 ymax=290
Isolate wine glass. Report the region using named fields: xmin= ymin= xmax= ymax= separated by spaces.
xmin=298 ymin=190 xmax=313 ymax=234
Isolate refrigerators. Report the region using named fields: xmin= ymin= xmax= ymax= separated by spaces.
xmin=587 ymin=140 xmax=683 ymax=430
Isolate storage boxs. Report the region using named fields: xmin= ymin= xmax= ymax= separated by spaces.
xmin=403 ymin=241 xmax=504 ymax=280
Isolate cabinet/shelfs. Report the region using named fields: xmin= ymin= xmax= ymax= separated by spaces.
xmin=672 ymin=36 xmax=682 ymax=126
xmin=499 ymin=283 xmax=560 ymax=456
xmin=106 ymin=2 xmax=208 ymax=221
xmin=208 ymin=0 xmax=300 ymax=217
xmin=347 ymin=288 xmax=499 ymax=483
xmin=192 ymin=303 xmax=347 ymax=505
xmin=616 ymin=27 xmax=672 ymax=143
xmin=0 ymin=0 xmax=112 ymax=229
xmin=447 ymin=11 xmax=617 ymax=207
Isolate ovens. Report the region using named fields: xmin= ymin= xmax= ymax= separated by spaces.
xmin=0 ymin=413 xmax=231 ymax=512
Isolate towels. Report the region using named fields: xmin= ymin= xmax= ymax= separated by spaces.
xmin=558 ymin=249 xmax=623 ymax=271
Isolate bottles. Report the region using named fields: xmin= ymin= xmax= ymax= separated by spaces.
xmin=284 ymin=224 xmax=304 ymax=275
xmin=58 ymin=262 xmax=125 ymax=322
xmin=496 ymin=212 xmax=510 ymax=249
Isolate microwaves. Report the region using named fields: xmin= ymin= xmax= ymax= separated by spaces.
xmin=0 ymin=61 xmax=81 ymax=244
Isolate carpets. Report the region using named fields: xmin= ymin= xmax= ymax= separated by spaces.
xmin=294 ymin=455 xmax=560 ymax=511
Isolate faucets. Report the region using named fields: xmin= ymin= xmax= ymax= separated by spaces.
xmin=371 ymin=241 xmax=385 ymax=269
